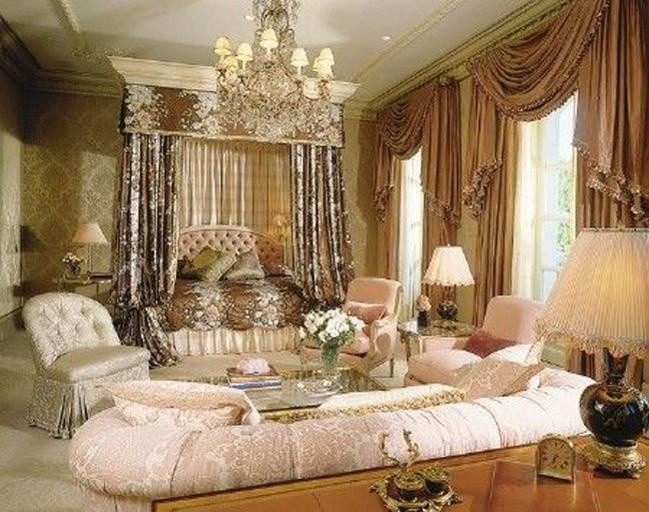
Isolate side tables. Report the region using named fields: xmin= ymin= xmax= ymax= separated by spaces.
xmin=397 ymin=317 xmax=475 ymax=365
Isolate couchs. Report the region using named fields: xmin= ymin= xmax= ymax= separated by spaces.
xmin=66 ymin=365 xmax=597 ymax=512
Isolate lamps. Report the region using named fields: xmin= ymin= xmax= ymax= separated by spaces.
xmin=532 ymin=226 xmax=648 ymax=479
xmin=73 ymin=222 xmax=107 ymax=272
xmin=214 ymin=1 xmax=335 ymax=106
xmin=422 ymin=245 xmax=475 ymax=328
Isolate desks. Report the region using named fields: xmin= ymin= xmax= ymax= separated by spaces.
xmin=151 ymin=437 xmax=649 ymax=511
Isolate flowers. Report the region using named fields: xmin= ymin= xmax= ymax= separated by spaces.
xmin=299 ymin=307 xmax=368 ymax=362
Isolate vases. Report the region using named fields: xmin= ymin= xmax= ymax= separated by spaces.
xmin=322 ymin=344 xmax=341 ymax=379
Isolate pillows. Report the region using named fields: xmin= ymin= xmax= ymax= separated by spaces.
xmin=315 ymin=383 xmax=466 ymax=416
xmin=99 ymin=376 xmax=261 ymax=428
xmin=181 ymin=244 xmax=237 ymax=282
xmin=223 ymin=247 xmax=265 ymax=281
xmin=453 ymin=342 xmax=545 ymax=402
xmin=463 ymin=328 xmax=515 ymax=358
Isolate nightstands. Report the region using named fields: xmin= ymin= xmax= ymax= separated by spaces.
xmin=64 ymin=274 xmax=112 ymax=302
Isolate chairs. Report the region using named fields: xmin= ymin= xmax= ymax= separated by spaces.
xmin=402 ymin=296 xmax=532 ymax=387
xmin=300 ymin=276 xmax=403 ymax=377
xmin=23 ymin=292 xmax=151 ymax=438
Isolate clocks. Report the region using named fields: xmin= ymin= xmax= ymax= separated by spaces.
xmin=535 ymin=434 xmax=576 ymax=482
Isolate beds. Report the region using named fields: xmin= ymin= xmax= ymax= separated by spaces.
xmin=159 ymin=223 xmax=301 ymax=356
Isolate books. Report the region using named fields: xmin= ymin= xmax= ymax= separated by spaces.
xmin=223 ymin=364 xmax=282 ymax=392
